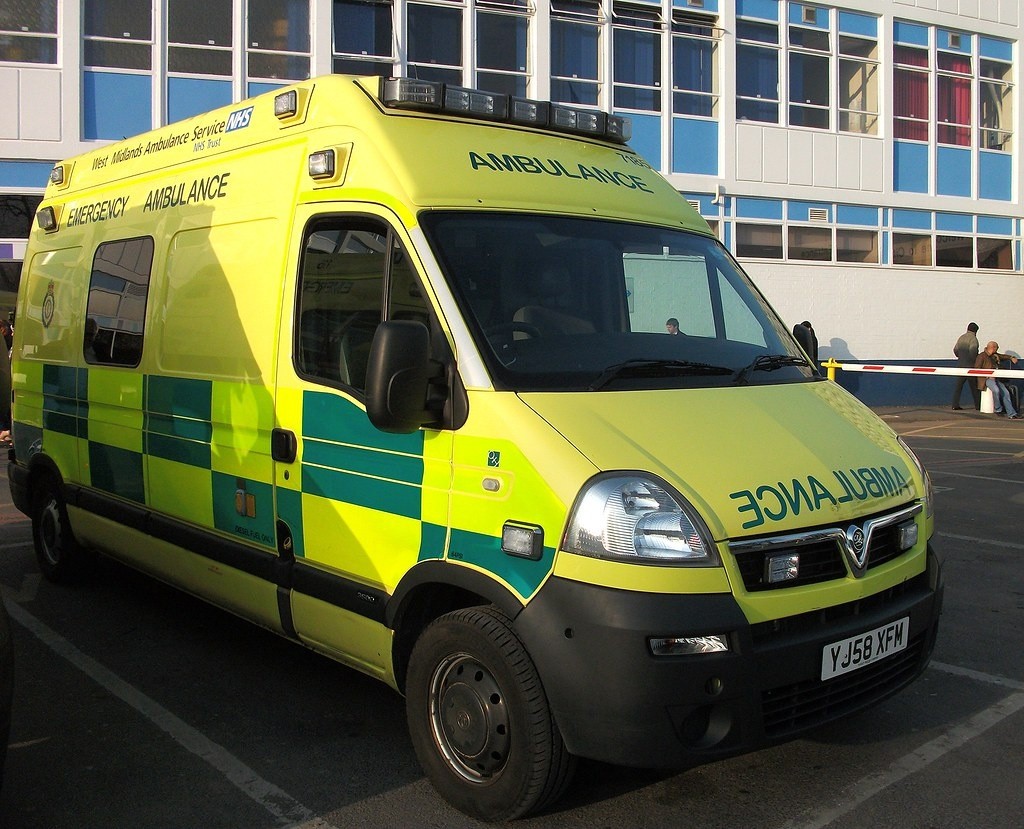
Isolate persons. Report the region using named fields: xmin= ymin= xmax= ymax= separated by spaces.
xmin=83 ymin=317 xmax=98 ymax=363
xmin=975 ymin=341 xmax=1023 ymax=419
xmin=0 ymin=319 xmax=15 ymax=447
xmin=666 ymin=318 xmax=687 ymax=336
xmin=801 ymin=321 xmax=819 ymax=369
xmin=951 ymin=322 xmax=982 ymax=410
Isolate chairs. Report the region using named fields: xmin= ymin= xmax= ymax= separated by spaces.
xmin=512 ymin=305 xmax=599 ymax=342
xmin=338 ymin=313 xmax=433 ymax=390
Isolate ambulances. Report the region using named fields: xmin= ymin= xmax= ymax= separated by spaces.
xmin=9 ymin=73 xmax=946 ymax=825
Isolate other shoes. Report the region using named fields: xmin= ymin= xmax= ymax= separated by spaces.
xmin=1009 ymin=413 xmax=1023 ymax=419
xmin=995 ymin=411 xmax=1004 ymax=416
xmin=952 ymin=405 xmax=962 ymax=410
